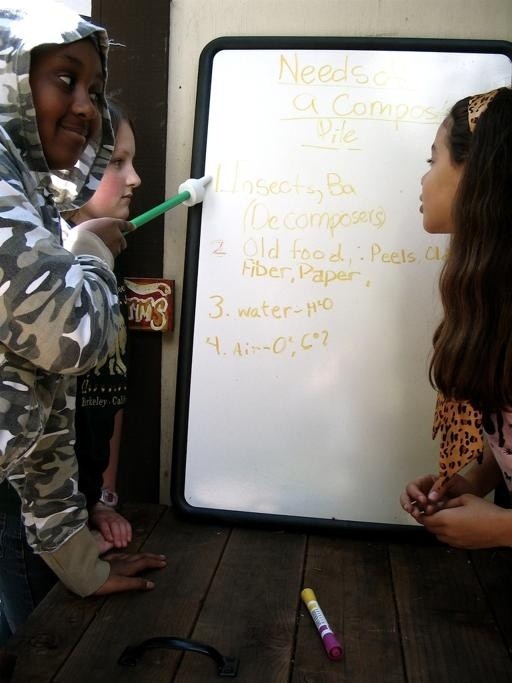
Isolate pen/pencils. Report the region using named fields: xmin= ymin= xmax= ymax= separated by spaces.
xmin=300 ymin=588 xmax=344 ymax=661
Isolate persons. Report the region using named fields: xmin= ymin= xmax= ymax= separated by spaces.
xmin=396 ymin=86 xmax=511 ymax=553
xmin=2 ymin=3 xmax=172 ymax=601
xmin=1 ymin=97 xmax=142 ymax=635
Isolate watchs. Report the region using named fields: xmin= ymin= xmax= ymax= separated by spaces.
xmin=99 ymin=485 xmax=121 ymax=509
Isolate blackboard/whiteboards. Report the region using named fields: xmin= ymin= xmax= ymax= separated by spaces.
xmin=170 ymin=35 xmax=512 ymax=534
